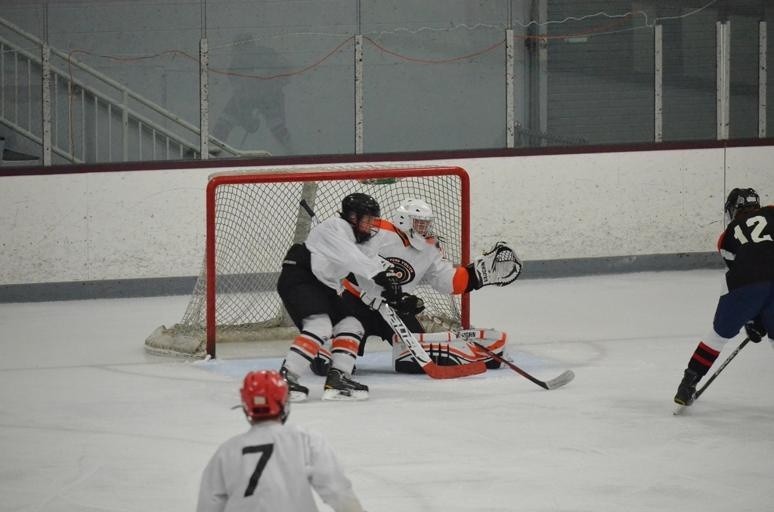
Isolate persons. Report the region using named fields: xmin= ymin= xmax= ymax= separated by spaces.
xmin=275 ymin=193 xmax=426 ymax=402
xmin=206 ymin=32 xmax=292 ymax=156
xmin=671 ymin=187 xmax=773 ymax=415
xmin=195 ymin=366 xmax=367 ymax=511
xmin=309 ymin=196 xmax=523 ymax=377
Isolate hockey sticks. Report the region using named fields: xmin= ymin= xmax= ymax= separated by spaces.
xmin=423 ymin=309 xmax=575 ymax=390
xmin=300 ymin=199 xmax=487 ymax=379
xmin=673 ymin=337 xmax=750 ymax=416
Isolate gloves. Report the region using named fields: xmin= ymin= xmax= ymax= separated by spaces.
xmin=372 ymin=270 xmax=425 ymax=314
xmin=745 ymin=321 xmax=761 ymax=343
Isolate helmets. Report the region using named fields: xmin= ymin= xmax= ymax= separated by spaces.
xmin=240 ymin=369 xmax=289 ymax=424
xmin=342 ymin=193 xmax=382 ymax=244
xmin=725 ymin=188 xmax=760 ymax=222
xmin=393 ymin=198 xmax=436 ymax=251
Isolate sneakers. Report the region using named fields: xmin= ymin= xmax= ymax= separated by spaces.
xmin=674 ymin=385 xmax=696 ymax=406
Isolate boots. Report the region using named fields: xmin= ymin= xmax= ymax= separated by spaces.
xmin=279 ymin=360 xmax=308 ymax=394
xmin=325 ymin=359 xmax=368 ymax=396
